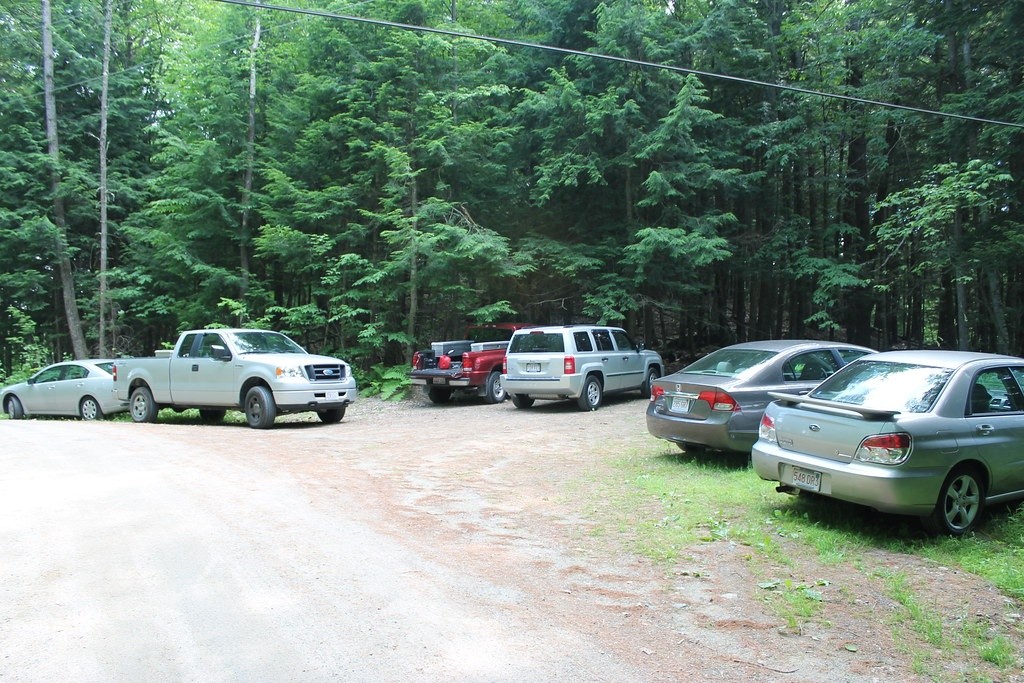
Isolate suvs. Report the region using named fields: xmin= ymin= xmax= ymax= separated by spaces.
xmin=499 ymin=324 xmax=665 ymax=413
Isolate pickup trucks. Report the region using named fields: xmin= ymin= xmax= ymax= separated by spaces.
xmin=404 ymin=323 xmax=540 ymax=404
xmin=109 ymin=327 xmax=357 ymax=429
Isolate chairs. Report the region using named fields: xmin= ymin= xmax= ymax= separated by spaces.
xmin=971 ymin=384 xmax=990 ymax=413
xmin=71 ymin=373 xmax=82 ymax=379
xmin=799 ymin=362 xmax=822 ymax=379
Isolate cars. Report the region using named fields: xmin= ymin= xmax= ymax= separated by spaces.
xmin=647 ymin=334 xmax=880 ymax=458
xmin=751 ymin=349 xmax=1024 ymax=539
xmin=0 ymin=358 xmax=132 ymax=421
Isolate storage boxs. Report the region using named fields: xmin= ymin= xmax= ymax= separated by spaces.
xmin=471 ymin=341 xmax=509 ymax=352
xmin=431 ymin=340 xmax=474 ymax=357
